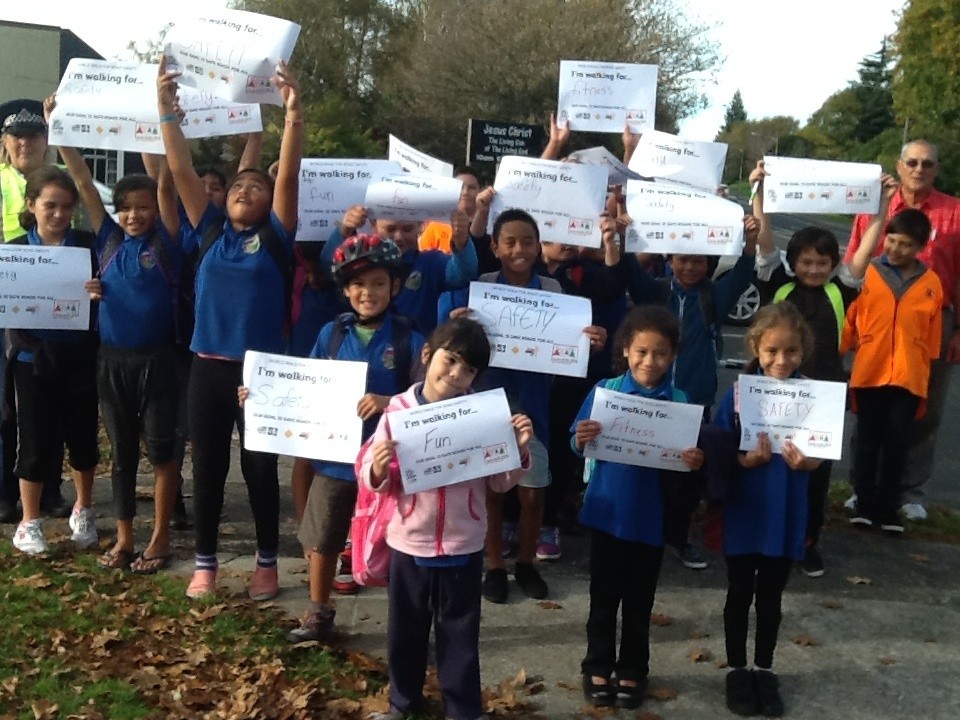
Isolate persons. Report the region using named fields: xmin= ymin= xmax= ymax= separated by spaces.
xmin=351 ymin=318 xmax=534 ymax=720
xmin=713 ymin=302 xmax=822 ymax=716
xmin=237 ymin=229 xmax=427 ymax=643
xmin=570 ymin=306 xmax=705 ymax=708
xmin=0 ymin=51 xmax=960 ymax=604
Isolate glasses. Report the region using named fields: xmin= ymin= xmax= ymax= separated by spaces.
xmin=902 ymin=159 xmax=938 ymax=169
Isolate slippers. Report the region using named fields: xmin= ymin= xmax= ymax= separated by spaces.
xmin=95 ymin=549 xmax=135 ymax=569
xmin=131 ymin=548 xmax=170 ymax=574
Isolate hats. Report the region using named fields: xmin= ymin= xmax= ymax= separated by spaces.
xmin=0 ymin=99 xmax=49 ymax=136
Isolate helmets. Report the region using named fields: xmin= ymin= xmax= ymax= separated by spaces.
xmin=330 ymin=233 xmax=401 ymax=283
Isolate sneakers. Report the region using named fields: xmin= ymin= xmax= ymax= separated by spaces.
xmin=13 ymin=518 xmax=49 ymax=555
xmin=500 ymin=523 xmax=514 ymax=556
xmin=536 ymin=525 xmax=563 ymax=559
xmin=68 ymin=503 xmax=98 ymax=550
xmin=332 ymin=546 xmax=363 ymax=595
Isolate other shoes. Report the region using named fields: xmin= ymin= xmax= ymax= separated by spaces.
xmin=849 ymin=501 xmax=873 ymax=526
xmin=248 ymin=565 xmax=279 ymax=600
xmin=582 ymin=670 xmax=612 ymax=705
xmin=674 ymin=547 xmax=708 ymax=568
xmin=290 ymin=607 xmax=336 ymax=640
xmin=880 ymin=506 xmax=904 ymax=532
xmin=514 ymin=562 xmax=549 ymax=599
xmin=371 ymin=705 xmax=414 ymax=720
xmin=483 ymin=568 xmax=509 ymax=603
xmin=40 ymin=485 xmax=69 ymax=516
xmin=802 ymin=547 xmax=824 ymax=577
xmin=750 ymin=666 xmax=784 ymax=717
xmin=186 ymin=567 xmax=217 ymax=601
xmin=725 ymin=668 xmax=756 ymax=717
xmin=0 ymin=498 xmax=18 ymax=522
xmin=613 ymin=677 xmax=646 ymax=707
xmin=169 ymin=498 xmax=188 ymax=527
